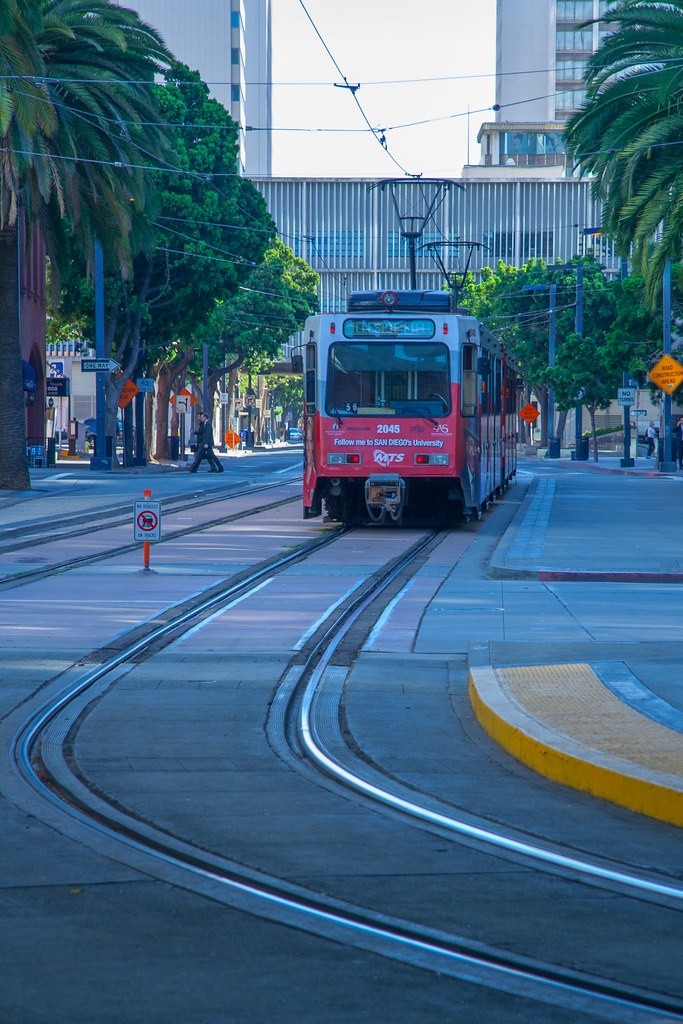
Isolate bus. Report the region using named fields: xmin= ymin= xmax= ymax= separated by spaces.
xmin=292 ymin=289 xmax=525 ymax=530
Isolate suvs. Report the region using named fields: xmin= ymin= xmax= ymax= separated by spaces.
xmin=83 ymin=417 xmax=137 ymax=450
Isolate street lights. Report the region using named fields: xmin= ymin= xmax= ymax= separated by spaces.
xmin=547 ymin=263 xmax=584 ymax=461
xmin=523 ymin=284 xmax=557 ymax=460
xmin=583 ymin=226 xmax=677 ymax=473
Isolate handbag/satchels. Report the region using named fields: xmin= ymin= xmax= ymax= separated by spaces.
xmin=645 ymin=436 xmax=647 ymax=441
xmin=199 ymin=447 xmax=214 ymax=458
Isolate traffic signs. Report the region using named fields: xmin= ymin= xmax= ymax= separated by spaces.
xmin=80 ymin=358 xmax=120 ymax=374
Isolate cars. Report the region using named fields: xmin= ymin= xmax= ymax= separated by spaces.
xmin=289 ymin=428 xmax=304 ymax=440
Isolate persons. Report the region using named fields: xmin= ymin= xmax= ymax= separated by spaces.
xmin=461 ymin=433 xmax=477 ymax=501
xmin=186 ymin=412 xmax=224 ymax=473
xmin=646 ymin=421 xmax=658 ymax=459
xmin=671 ymin=416 xmax=683 ymax=470
xmin=304 ymin=417 xmax=317 ymax=506
xmin=190 ymin=412 xmax=217 ymax=473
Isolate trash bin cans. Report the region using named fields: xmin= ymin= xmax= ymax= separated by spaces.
xmin=245 ymin=431 xmax=254 ymax=447
xmin=166 ymin=436 xmax=179 ymax=461
xmin=548 ymin=436 xmax=561 ymax=458
xmin=93 ymin=435 xmax=113 ymax=458
xmin=658 ymin=437 xmax=677 ymax=470
xmin=575 ymin=436 xmax=589 ymax=460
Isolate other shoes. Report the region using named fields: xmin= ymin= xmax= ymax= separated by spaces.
xmin=208 ymin=468 xmax=217 ymax=472
xmin=679 ymin=464 xmax=683 ymax=470
xmin=646 ymin=456 xmax=650 ymax=459
xmin=216 ymin=468 xmax=224 ymax=473
xmin=186 ymin=465 xmax=193 ymax=471
xmin=191 ymin=470 xmax=197 ymax=473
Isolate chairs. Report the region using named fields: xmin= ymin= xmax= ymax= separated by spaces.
xmin=32 ymin=446 xmax=46 ymax=469
